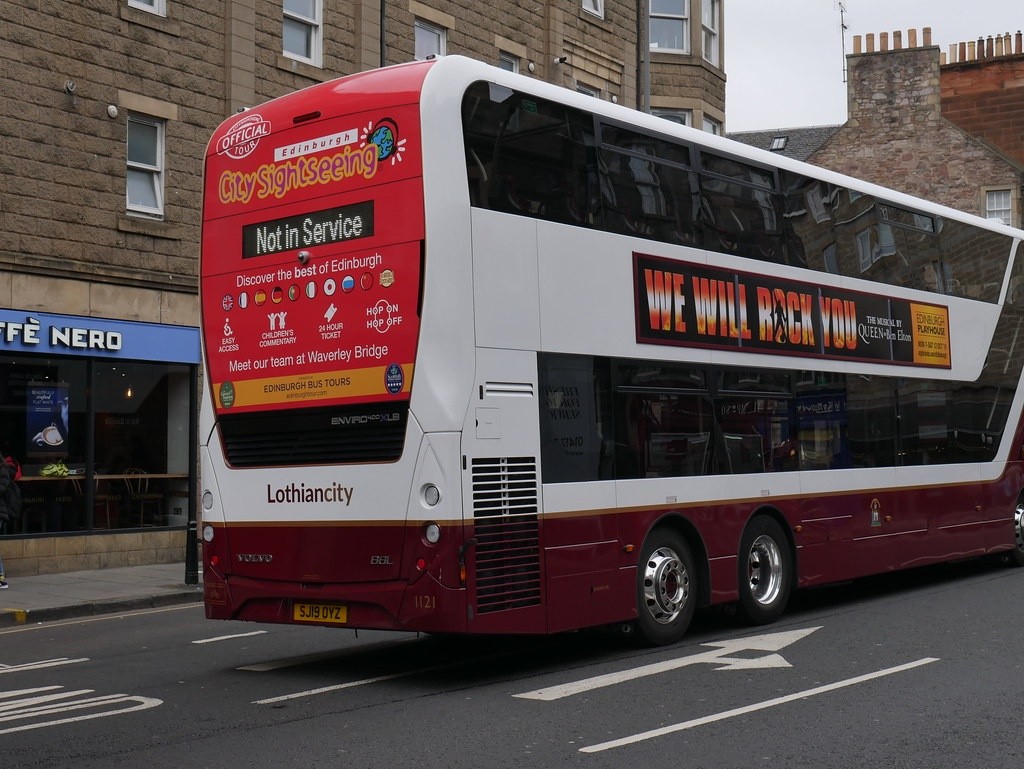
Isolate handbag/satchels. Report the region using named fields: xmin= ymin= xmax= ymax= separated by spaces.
xmin=39 ymin=462 xmax=68 ymax=477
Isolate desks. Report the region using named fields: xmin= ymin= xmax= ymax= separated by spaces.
xmin=14 ymin=474 xmax=189 ymax=482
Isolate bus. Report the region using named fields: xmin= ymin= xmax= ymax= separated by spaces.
xmin=197 ymin=53 xmax=1024 ymax=645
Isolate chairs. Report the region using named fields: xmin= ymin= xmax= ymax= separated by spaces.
xmin=72 ymin=468 xmax=110 ymax=533
xmin=124 ymin=469 xmax=164 ymax=525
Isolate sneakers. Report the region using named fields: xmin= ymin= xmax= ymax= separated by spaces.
xmin=0 ymin=581 xmax=8 ymax=589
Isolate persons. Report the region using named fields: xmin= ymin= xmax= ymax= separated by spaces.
xmin=0 ymin=453 xmax=22 ymax=589
